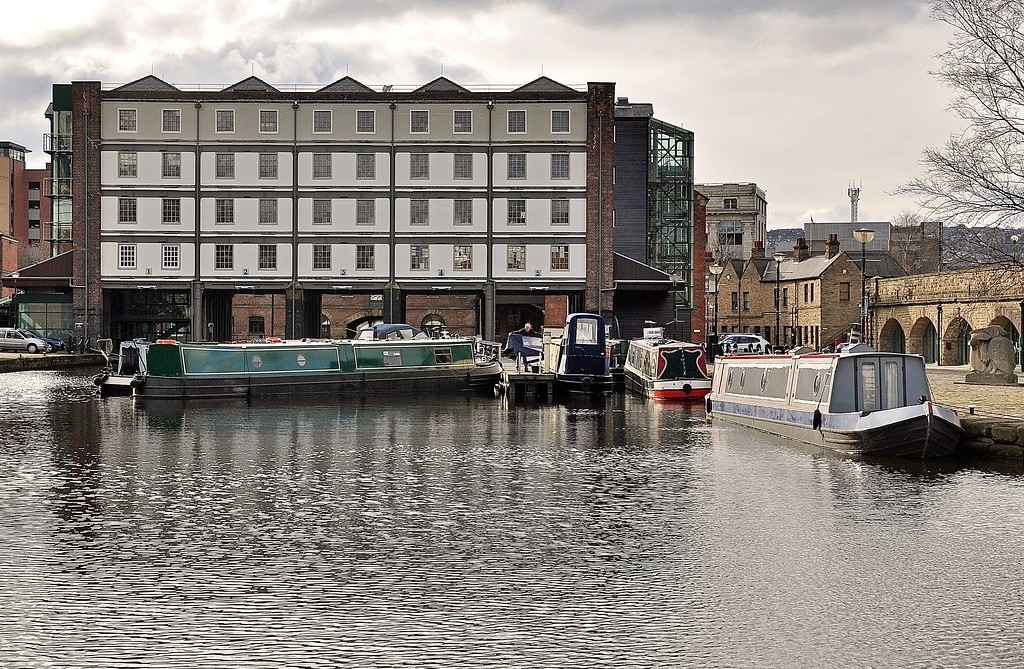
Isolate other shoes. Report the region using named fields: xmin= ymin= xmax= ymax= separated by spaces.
xmin=525 ymin=368 xmax=531 ymax=371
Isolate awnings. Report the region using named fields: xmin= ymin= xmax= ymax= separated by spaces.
xmin=0 ymin=249 xmax=73 ymax=287
xmin=613 ymin=250 xmax=685 ymax=291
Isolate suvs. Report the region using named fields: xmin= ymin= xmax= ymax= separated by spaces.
xmin=718 ymin=334 xmax=770 ymax=356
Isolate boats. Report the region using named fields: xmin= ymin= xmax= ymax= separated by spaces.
xmin=704 ymin=353 xmax=964 ymax=477
xmin=621 ymin=339 xmax=712 ymax=401
xmin=553 ymin=312 xmax=620 ymax=406
xmin=92 ymin=338 xmax=505 ymax=401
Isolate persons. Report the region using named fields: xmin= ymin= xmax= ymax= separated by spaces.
xmin=747 ymin=342 xmax=761 ymax=352
xmin=507 ymin=323 xmax=543 ymax=373
xmin=725 ymin=342 xmax=738 ymax=353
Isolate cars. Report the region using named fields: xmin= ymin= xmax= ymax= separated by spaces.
xmin=0 ymin=328 xmax=52 ymax=354
xmin=26 ymin=330 xmax=64 ymax=354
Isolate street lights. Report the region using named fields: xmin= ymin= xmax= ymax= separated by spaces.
xmin=709 ymin=263 xmax=724 ymax=357
xmin=853 ymin=227 xmax=876 ymax=343
xmin=771 ymin=252 xmax=785 ymax=345
